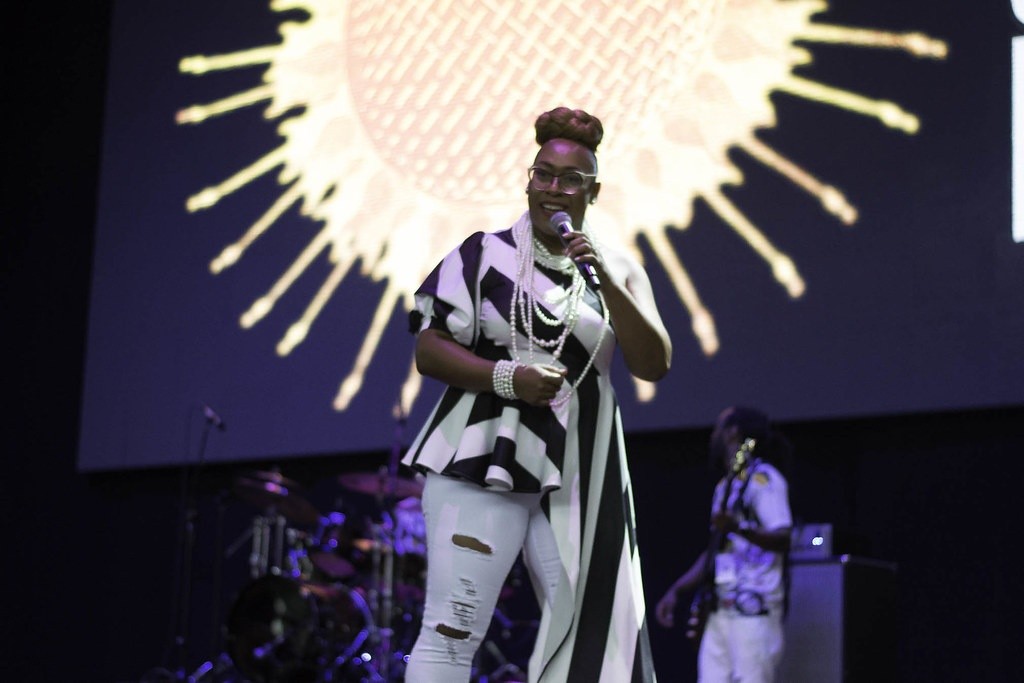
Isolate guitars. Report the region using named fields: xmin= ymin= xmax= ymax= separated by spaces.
xmin=684 ymin=435 xmax=759 ymax=645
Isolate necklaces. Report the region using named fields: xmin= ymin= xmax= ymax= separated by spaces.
xmin=512 ymin=210 xmax=610 ymax=406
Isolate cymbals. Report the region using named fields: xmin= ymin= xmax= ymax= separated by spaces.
xmin=339 ymin=473 xmax=423 ymax=497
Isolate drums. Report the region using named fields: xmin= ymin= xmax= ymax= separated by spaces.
xmin=228 ymin=572 xmax=372 ymax=683
xmin=306 ymin=513 xmax=363 ymax=578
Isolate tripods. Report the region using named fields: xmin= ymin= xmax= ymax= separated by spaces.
xmin=191 ymin=500 xmax=411 ymax=683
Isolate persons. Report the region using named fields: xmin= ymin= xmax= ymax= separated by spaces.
xmin=657 ymin=406 xmax=793 ymax=683
xmin=269 ymin=465 xmax=533 ymax=683
xmin=403 ymin=105 xmax=672 ymax=683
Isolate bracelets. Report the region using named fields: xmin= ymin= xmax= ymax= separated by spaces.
xmin=491 ymin=360 xmax=524 ymax=399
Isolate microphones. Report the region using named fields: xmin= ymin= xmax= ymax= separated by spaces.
xmin=550 ymin=211 xmax=600 ymax=290
xmin=199 ymin=402 xmax=227 ymax=432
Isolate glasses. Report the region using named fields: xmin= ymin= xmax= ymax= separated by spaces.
xmin=528 ymin=166 xmax=597 ymax=195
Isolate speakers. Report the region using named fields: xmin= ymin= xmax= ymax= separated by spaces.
xmin=773 ymin=554 xmax=899 ymax=683
